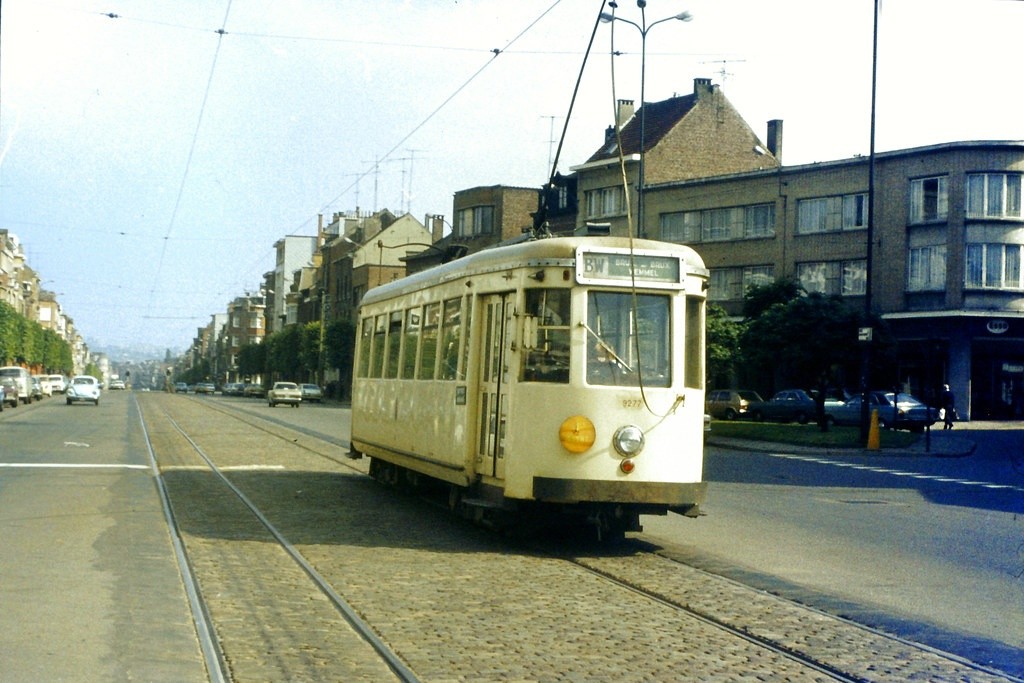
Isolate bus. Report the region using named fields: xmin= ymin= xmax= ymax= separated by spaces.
xmin=348 ymin=219 xmax=706 ymax=551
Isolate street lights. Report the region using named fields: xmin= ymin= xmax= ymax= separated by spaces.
xmin=600 ymin=12 xmax=693 ymax=232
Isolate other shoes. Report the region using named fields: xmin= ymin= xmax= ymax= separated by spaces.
xmin=944 ymin=425 xmax=947 ymax=429
xmin=949 ymin=424 xmax=953 ymax=429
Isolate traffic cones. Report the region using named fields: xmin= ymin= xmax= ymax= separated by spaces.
xmin=863 ymin=408 xmax=884 ymax=450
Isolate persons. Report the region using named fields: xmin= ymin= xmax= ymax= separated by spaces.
xmin=941 ymin=384 xmax=954 ymax=429
xmin=541 ymin=321 xmax=570 ymax=383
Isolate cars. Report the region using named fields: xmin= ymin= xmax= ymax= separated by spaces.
xmin=704 ymin=389 xmax=765 ymax=420
xmin=187 ymin=382 xmax=216 ymax=395
xmin=65 ymin=375 xmax=104 ymax=405
xmin=748 ymin=388 xmax=819 ymax=424
xmin=827 ymin=391 xmax=939 ymax=433
xmin=245 ymin=384 xmax=265 ymax=398
xmin=267 ymin=381 xmax=302 ymax=409
xmin=0 ymin=365 xmax=69 ymax=413
xmin=297 ymin=383 xmax=322 ymax=404
xmin=108 ymin=380 xmax=126 ymax=390
xmin=175 ymin=382 xmax=188 ymax=394
xmin=221 ymin=382 xmax=246 ymax=396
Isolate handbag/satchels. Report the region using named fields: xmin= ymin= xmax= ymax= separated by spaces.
xmin=939 ymin=408 xmax=945 ymax=420
xmin=952 ymin=408 xmax=958 ymax=420
